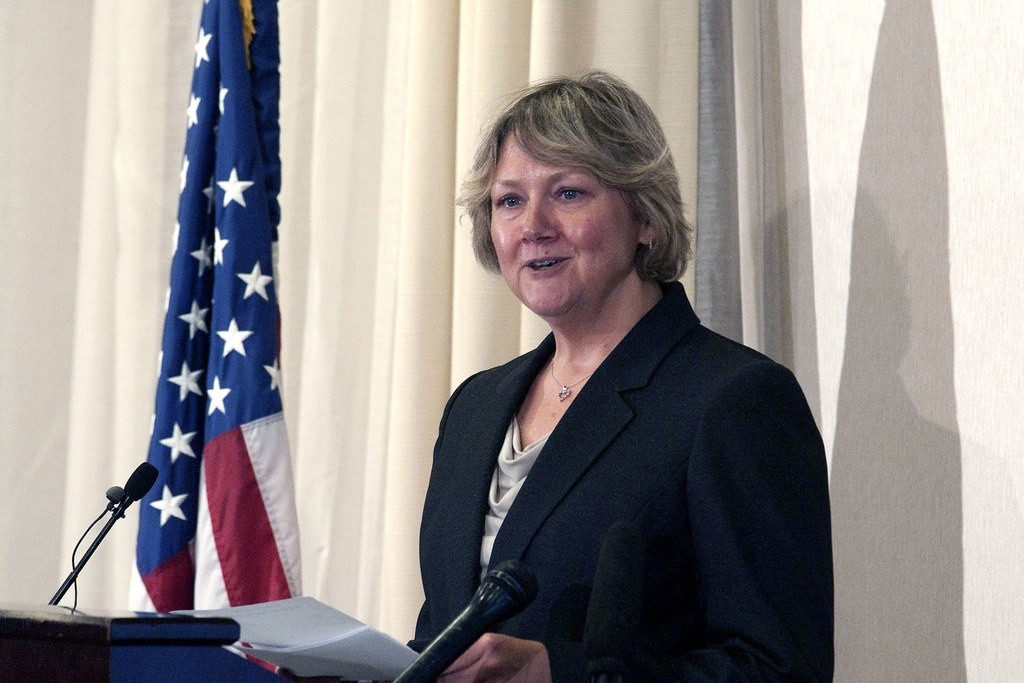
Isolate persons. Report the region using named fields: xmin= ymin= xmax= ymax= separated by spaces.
xmin=408 ymin=71 xmax=835 ymax=683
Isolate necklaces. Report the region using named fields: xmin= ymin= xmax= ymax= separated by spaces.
xmin=551 ymin=360 xmax=591 ymax=401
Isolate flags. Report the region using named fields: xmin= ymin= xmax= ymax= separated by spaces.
xmin=130 ymin=0 xmax=302 ymax=675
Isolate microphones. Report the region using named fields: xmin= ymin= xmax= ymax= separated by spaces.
xmin=394 ymin=558 xmax=541 ymax=683
xmin=49 ymin=462 xmax=160 ymax=605
